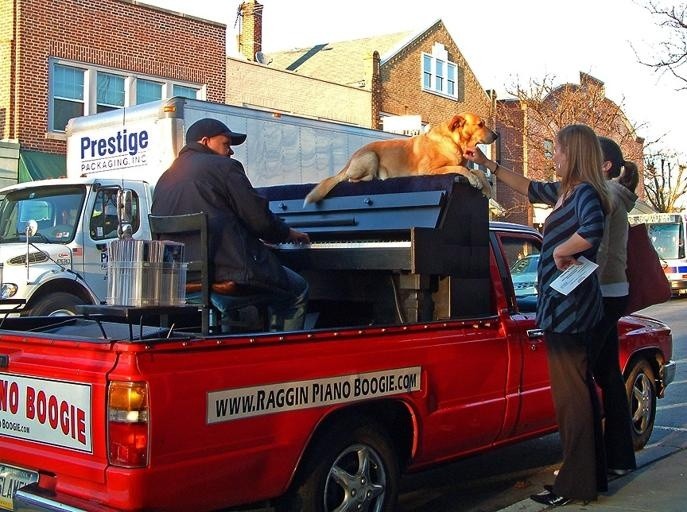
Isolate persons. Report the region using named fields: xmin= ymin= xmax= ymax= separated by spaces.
xmin=464 ymin=123 xmax=609 ymax=506
xmin=151 ymin=117 xmax=311 ymax=334
xmin=592 ymin=137 xmax=639 ymax=476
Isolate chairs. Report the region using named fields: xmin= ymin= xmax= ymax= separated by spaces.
xmin=148 ymin=211 xmax=285 ymax=335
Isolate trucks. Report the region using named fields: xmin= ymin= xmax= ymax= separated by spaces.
xmin=1 ymin=94 xmax=421 ymax=320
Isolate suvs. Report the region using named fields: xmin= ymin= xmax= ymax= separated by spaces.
xmin=510 ymin=253 xmax=541 ymax=309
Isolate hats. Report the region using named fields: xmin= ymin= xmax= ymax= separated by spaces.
xmin=185 ymin=118 xmax=247 ymax=146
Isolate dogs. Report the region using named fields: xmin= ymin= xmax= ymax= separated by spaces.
xmin=302 ymin=111 xmax=499 ymax=210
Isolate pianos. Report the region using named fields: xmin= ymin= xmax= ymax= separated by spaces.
xmin=252 ymin=173 xmax=489 ymax=325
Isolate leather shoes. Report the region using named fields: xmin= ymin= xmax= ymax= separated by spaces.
xmin=530 ymin=483 xmax=596 ymax=507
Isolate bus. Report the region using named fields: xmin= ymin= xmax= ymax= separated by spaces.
xmin=625 ymin=212 xmax=687 ymax=300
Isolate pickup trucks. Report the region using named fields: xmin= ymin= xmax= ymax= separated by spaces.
xmin=0 ymin=171 xmax=680 ymax=512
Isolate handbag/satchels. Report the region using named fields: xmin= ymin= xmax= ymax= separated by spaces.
xmin=623 ymin=221 xmax=672 ymax=317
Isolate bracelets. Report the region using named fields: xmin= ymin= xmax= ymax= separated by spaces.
xmin=488 ymin=160 xmax=499 ymax=175
